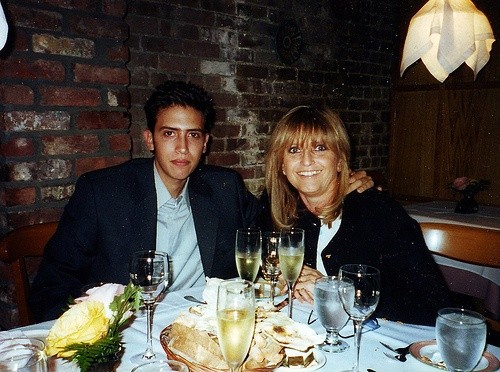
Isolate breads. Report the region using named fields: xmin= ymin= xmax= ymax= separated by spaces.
xmin=169 ymin=312 xmax=318 ymax=371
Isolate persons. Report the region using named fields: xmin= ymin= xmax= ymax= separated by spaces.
xmin=250 ymin=104 xmax=459 ymax=327
xmin=26 ymin=78 xmax=375 ymax=323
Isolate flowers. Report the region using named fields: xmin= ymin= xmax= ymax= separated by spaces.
xmin=446 ymin=175 xmax=491 ymax=192
xmin=45 ymin=279 xmax=141 ymax=372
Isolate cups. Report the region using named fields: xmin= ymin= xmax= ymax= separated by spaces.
xmin=435 ymin=308 xmax=487 ymax=372
xmin=0 ymin=338 xmax=48 ymax=372
xmin=216 ymin=279 xmax=255 ymax=371
xmin=130 ymin=360 xmax=189 ymax=372
xmin=235 ymin=227 xmax=262 ymax=283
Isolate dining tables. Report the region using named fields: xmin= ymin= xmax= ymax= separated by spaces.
xmin=403 ymin=200 xmax=500 ymax=286
xmin=0 ymin=277 xmax=500 ymax=372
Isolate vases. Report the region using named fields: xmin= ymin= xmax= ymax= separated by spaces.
xmin=454 ymin=191 xmax=479 ymax=215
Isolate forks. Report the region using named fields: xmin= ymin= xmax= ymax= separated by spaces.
xmin=379 ymin=338 xmax=436 ymax=363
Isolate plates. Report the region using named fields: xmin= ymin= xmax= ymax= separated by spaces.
xmin=409 ymin=341 xmax=500 ymax=372
xmin=229 ymin=278 xmax=289 ymax=307
xmin=272 ymin=348 xmax=326 ymax=372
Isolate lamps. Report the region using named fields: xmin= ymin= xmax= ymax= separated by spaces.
xmin=400 ymin=0 xmax=496 ymax=84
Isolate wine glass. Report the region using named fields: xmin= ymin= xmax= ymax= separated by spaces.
xmin=277 ymin=229 xmax=305 ymax=319
xmin=312 ymin=276 xmax=355 ymax=353
xmin=261 ymin=230 xmax=282 ymax=312
xmin=130 ymin=250 xmax=169 ymax=366
xmin=337 ymin=264 xmax=380 ymax=372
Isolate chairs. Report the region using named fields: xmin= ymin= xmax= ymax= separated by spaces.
xmin=0 ymin=222 xmax=57 ymax=323
xmin=418 ymin=224 xmax=500 ymax=333
